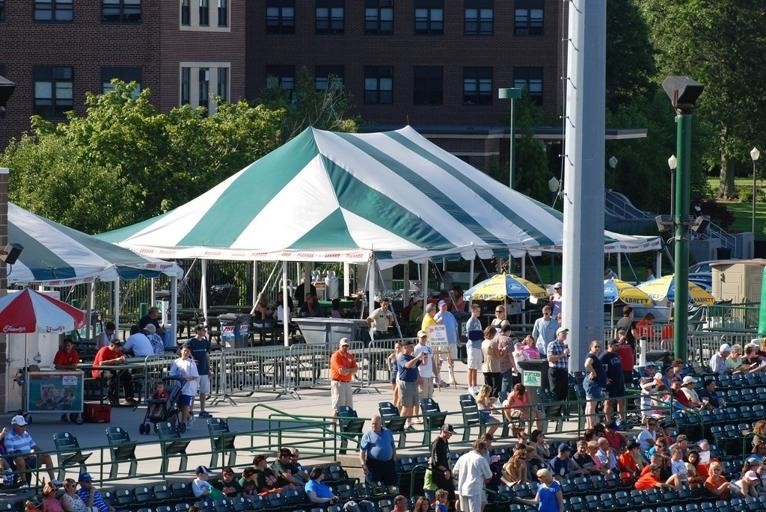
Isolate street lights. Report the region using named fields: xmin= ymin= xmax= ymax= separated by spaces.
xmin=548 ymin=176 xmax=559 ymax=210
xmin=666 ymin=154 xmax=677 ymax=219
xmin=749 ymin=147 xmax=761 ymax=232
xmin=608 ymin=156 xmax=619 ymax=190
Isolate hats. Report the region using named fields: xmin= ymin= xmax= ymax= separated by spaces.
xmin=280 ymin=447 xmax=295 ymax=457
xmin=559 ymin=443 xmax=573 ymax=452
xmin=664 ymin=364 xmax=673 ymax=372
xmin=645 ymin=361 xmax=655 ymax=369
xmin=194 ymin=324 xmax=208 ymax=332
xmin=677 ymin=434 xmax=688 ymax=441
xmin=608 ymin=338 xmax=621 ymax=345
xmin=683 ymin=376 xmax=698 ymax=384
xmin=752 ymin=339 xmax=760 ymax=346
xmin=79 ymin=472 xmax=92 ymax=481
xmin=438 ymin=300 xmax=447 ymax=307
xmin=413 ymin=296 xmax=424 ymax=304
xmin=720 ymin=343 xmax=734 ymax=352
xmin=746 ymin=469 xmax=759 ymax=480
xmin=253 ymin=454 xmax=269 ymax=464
xmin=196 ymin=465 xmax=212 ymax=474
xmin=403 ymin=340 xmax=414 ymax=345
xmin=442 ymin=423 xmax=457 ymax=435
xmin=111 ymin=338 xmax=122 ymax=346
xmin=628 ymin=439 xmax=640 ymax=450
xmin=748 ymin=457 xmax=761 ymax=466
xmin=340 ymin=337 xmax=350 ymax=346
xmin=554 ymin=282 xmax=562 ymax=288
xmin=556 ymin=327 xmax=569 ymax=336
xmin=11 ymin=414 xmax=27 ymax=426
xmin=417 ymin=329 xmax=428 ymax=337
xmin=143 ymin=323 xmax=156 ymax=333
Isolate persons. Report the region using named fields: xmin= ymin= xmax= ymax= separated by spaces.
xmin=238 ymin=467 xmax=259 ymax=497
xmin=53 ymin=338 xmax=80 ymax=423
xmin=0 ymin=423 xmax=10 ymax=471
xmin=3 ymin=415 xmax=53 ymax=483
xmin=365 ymin=298 xmax=394 ymax=366
xmin=502 ymin=383 xmax=543 ymax=434
xmin=387 ymin=342 xmax=402 ymax=406
xmin=421 ymin=303 xmax=448 ymax=388
xmin=491 ymin=305 xmax=510 ymax=334
xmin=465 ymin=303 xmax=484 ymax=396
xmin=97 ymin=322 xmax=115 ymax=346
xmin=59 ymin=478 xmax=86 ymax=512
xmin=390 ymin=494 xmax=409 ymax=512
xmin=635 ymin=313 xmax=655 ymax=338
xmin=500 ymin=424 xmax=639 ymax=512
xmin=119 ymin=325 xmax=153 ymax=393
xmin=617 ymin=306 xmax=638 ymax=350
xmin=481 ymin=433 xmax=492 ymax=512
xmin=192 ymin=466 xmax=213 ymax=498
xmin=414 ymin=330 xmax=440 ymax=399
xmin=409 ymin=285 xmax=466 ymax=323
xmin=253 ymin=455 xmax=280 ymax=492
xmin=521 ymin=334 xmax=539 ymax=360
xmin=532 ymin=305 xmax=559 ymax=358
xmin=169 ymin=344 xmax=199 ymax=432
xmin=92 ymin=339 xmax=139 ymax=407
xmin=433 ymin=300 xmax=459 ymax=386
xmin=75 ymin=473 xmax=116 ymax=512
xmin=270 ymin=448 xmax=308 ymax=486
xmin=361 ymin=416 xmax=396 ymax=496
xmin=148 ymin=381 xmax=169 ymax=417
xmin=185 ymin=324 xmax=212 ymax=418
xmin=645 ymin=266 xmax=656 ymax=281
xmin=432 ymin=424 xmax=456 ymax=488
xmin=583 ymin=340 xmax=607 ymax=430
xmin=304 ymin=467 xmax=339 ymax=504
xmin=289 ymin=449 xmax=304 ymax=474
xmin=639 ymin=336 xmax=766 ymax=500
xmin=548 ymin=282 xmax=562 ymax=320
xmin=330 ymin=337 xmax=359 ymax=426
xmin=423 ymin=454 xmax=439 ymax=500
xmin=213 ymin=467 xmax=242 ymax=497
xmin=435 ymin=489 xmax=449 ymax=512
xmin=452 ymin=439 xmax=492 ymax=512
xmin=139 ymin=307 xmax=163 ymax=336
xmin=481 ymin=326 xmax=508 ymax=414
xmin=411 ymin=375 xmax=424 ymax=425
xmin=492 ymin=326 xmax=518 ymax=413
xmin=598 ymin=339 xmax=625 ymax=423
xmin=475 ymin=385 xmax=500 ymax=441
xmin=42 ymin=482 xmax=64 ymax=512
xmin=395 ymin=341 xmax=428 ymax=431
xmin=255 ymin=276 xmax=342 ymax=344
xmin=546 ymin=328 xmax=570 ymax=422
xmin=142 ymin=324 xmax=164 ymax=355
xmin=413 ymin=496 xmax=431 ymax=512
xmin=661 ymin=318 xmax=674 ymax=340
xmin=512 ymin=337 xmax=528 ymax=385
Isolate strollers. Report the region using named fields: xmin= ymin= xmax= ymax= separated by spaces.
xmin=138 ymin=375 xmax=188 ymax=435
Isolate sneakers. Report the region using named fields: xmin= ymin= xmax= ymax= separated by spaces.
xmin=20 ymin=484 xmax=29 ymax=492
xmin=199 ymin=411 xmax=212 ymax=418
xmin=186 ymin=417 xmax=196 ymax=432
xmin=124 ymin=398 xmax=137 ymax=404
xmin=52 ymin=479 xmax=64 ymax=487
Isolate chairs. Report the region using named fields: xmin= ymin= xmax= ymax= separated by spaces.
xmin=378 ymin=402 xmax=407 ymax=448
xmin=207 ymin=418 xmax=236 ymax=468
xmin=156 ymin=421 xmax=191 ymax=473
xmin=459 ymin=394 xmax=487 ymax=441
xmin=488 ymin=439 xmax=766 ymax=512
xmin=101 ymin=482 xmax=190 ymax=512
xmin=193 ymin=454 xmax=461 ymax=512
xmin=336 ymin=406 xmax=363 ymax=454
xmin=537 ymin=387 xmax=563 ymax=433
xmin=0 ymin=501 xmax=16 ymax=512
xmin=106 ymin=427 xmax=138 ymax=479
xmin=419 ymin=398 xmax=447 ymax=445
xmin=498 ymin=391 xmax=525 ymax=438
xmin=574 ymin=383 xmax=587 ymax=430
xmin=52 ymin=431 xmax=92 ymax=481
xmin=628 ymin=360 xmax=766 ymax=446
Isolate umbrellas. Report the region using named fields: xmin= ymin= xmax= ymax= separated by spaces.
xmin=463 ymin=272 xmax=550 ymax=319
xmin=635 ymin=273 xmax=716 ymax=324
xmin=0 ymin=287 xmax=87 ymax=412
xmin=604 ymin=276 xmax=652 ymax=339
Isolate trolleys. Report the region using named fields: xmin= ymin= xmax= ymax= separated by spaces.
xmin=13 ymin=365 xmax=85 ymax=425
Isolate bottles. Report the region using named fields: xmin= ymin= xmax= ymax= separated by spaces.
xmin=421 ymin=351 xmax=427 ymax=365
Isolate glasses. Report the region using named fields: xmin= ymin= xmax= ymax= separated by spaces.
xmin=86 ymin=480 xmax=93 ymax=483
xmin=648 ymin=422 xmax=656 ymax=425
xmin=647 ymin=365 xmax=655 ymax=368
xmin=51 ymin=488 xmax=58 ymax=491
xmin=543 ymin=312 xmax=549 ymax=315
xmin=656 ymin=443 xmax=664 ymax=448
xmin=714 ymin=468 xmax=721 ymax=471
xmin=595 ymin=345 xmax=600 ymax=348
xmin=496 ymin=310 xmax=503 ymax=312
xmin=736 ymin=352 xmax=741 ymax=355
xmin=71 ymin=483 xmax=77 ymax=487
